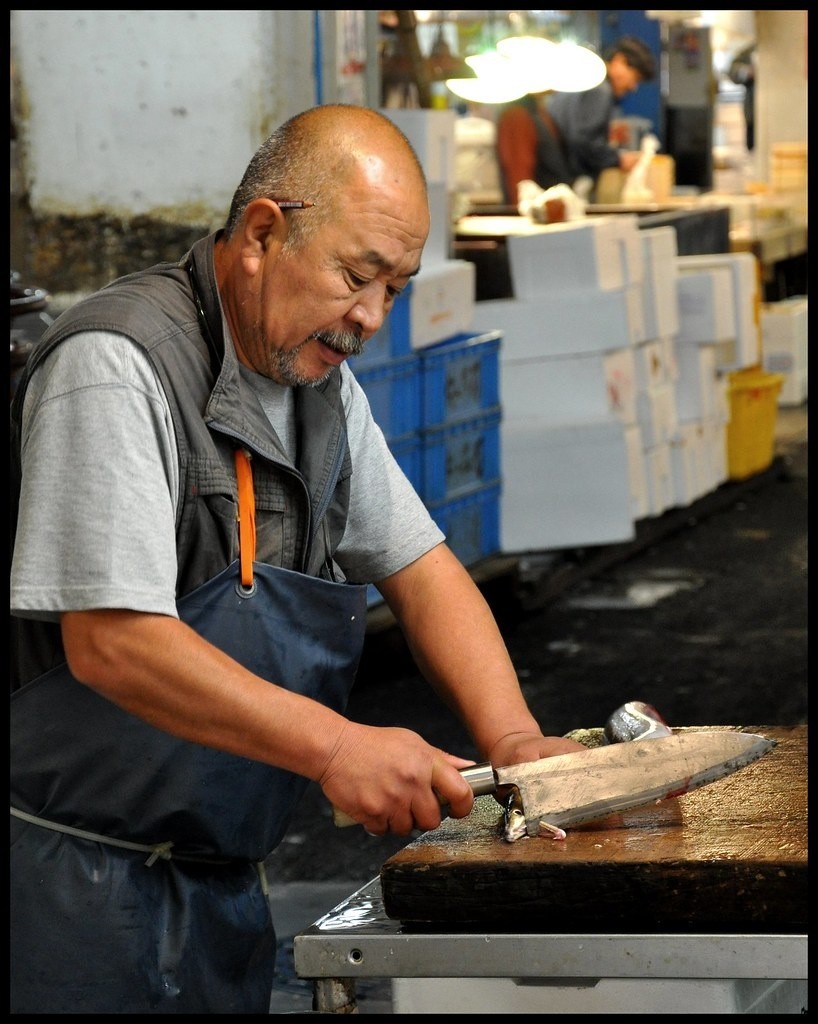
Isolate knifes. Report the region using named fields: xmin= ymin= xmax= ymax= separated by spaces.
xmin=332 ymin=729 xmax=780 ymax=839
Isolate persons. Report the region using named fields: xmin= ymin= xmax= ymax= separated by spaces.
xmin=729 ymin=41 xmax=754 ymax=151
xmin=9 ymin=103 xmax=594 ymax=1013
xmin=378 ymin=8 xmax=434 ymax=109
xmin=538 ymin=30 xmax=656 ymax=169
xmin=492 ymin=87 xmax=581 ymax=205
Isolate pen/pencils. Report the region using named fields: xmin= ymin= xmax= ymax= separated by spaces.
xmin=246 ymin=199 xmax=316 ymax=210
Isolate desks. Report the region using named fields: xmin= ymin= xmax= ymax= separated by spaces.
xmin=293 ymin=876 xmax=809 ymax=1016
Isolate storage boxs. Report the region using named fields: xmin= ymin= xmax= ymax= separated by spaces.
xmin=345 ymin=116 xmax=812 ymax=567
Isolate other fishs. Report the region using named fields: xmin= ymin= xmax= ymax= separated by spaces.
xmin=502 ymin=700 xmax=675 ymax=843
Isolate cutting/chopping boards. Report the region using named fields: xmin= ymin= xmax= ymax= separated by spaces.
xmin=379 ymin=724 xmax=809 ymax=928
